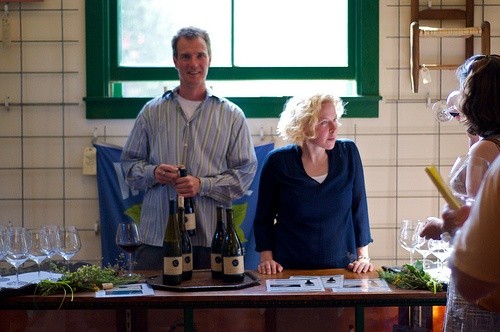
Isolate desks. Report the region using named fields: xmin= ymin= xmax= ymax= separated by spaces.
xmin=0 ymin=268 xmax=446 ymax=332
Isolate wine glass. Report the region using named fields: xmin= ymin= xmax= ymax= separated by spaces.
xmin=428 ymin=230 xmax=455 ymax=282
xmin=23 ymin=227 xmax=52 ymax=284
xmin=53 ymin=225 xmax=81 ymax=274
xmin=412 ymin=222 xmax=434 ymax=272
xmin=0 ymin=227 xmax=11 ymax=281
xmin=115 ymin=223 xmax=142 ymax=278
xmin=400 ymin=220 xmax=424 ymax=268
xmin=4 ymin=227 xmax=28 ymax=285
xmin=432 ymin=91 xmax=461 ymax=121
xmin=39 ymin=224 xmax=60 ymax=282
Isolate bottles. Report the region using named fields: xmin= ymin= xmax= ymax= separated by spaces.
xmin=210 ymin=205 xmax=225 ymax=279
xmin=163 ymin=199 xmax=183 ymax=287
xmin=221 ymin=209 xmax=245 ymax=283
xmin=178 ymin=166 xmax=197 ymax=235
xmin=177 ymin=206 xmax=193 ymax=281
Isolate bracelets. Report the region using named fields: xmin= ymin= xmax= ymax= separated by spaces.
xmin=357 ymin=256 xmax=370 ymax=260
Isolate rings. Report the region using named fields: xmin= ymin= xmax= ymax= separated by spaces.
xmin=163 ymin=171 xmax=167 ymax=176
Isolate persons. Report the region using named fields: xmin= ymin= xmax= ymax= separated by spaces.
xmin=254 ymin=92 xmax=375 ymax=275
xmin=418 ymin=55 xmax=500 ymax=332
xmin=121 ymin=27 xmax=259 ymax=272
